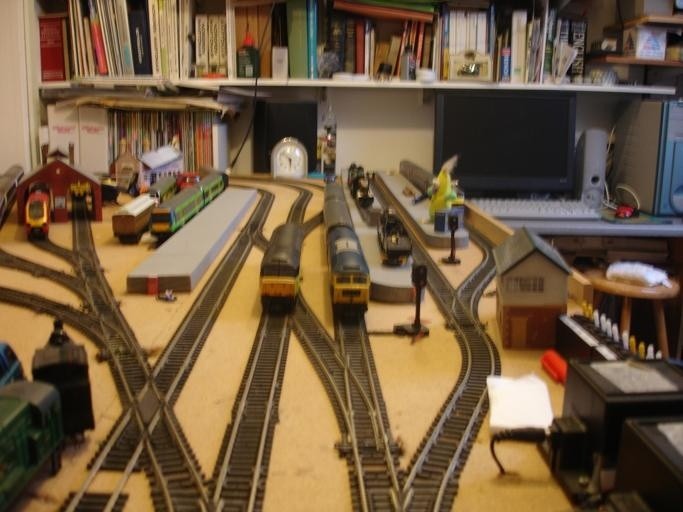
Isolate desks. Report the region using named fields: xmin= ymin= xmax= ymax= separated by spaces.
xmin=498 ymin=218 xmax=683 ymax=331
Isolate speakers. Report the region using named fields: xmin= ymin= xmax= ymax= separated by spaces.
xmin=574 ymin=129 xmax=607 ymax=210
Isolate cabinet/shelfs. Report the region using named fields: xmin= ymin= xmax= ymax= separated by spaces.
xmin=23 ymin=0 xmax=683 ymax=104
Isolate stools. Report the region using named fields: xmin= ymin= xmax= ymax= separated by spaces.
xmin=583 ymin=265 xmax=681 ymax=361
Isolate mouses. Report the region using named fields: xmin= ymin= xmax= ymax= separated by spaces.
xmin=615 ymin=206 xmax=639 ymax=219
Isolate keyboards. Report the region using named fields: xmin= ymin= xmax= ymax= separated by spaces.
xmin=465 ymin=197 xmax=602 ymax=221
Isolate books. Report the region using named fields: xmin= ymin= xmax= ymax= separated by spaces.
xmin=35 ymin=86 xmax=248 ymax=179
xmin=58 ymin=0 xmax=587 ymax=85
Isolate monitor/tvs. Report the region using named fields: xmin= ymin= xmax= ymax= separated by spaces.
xmin=433 ymin=90 xmax=576 ymax=200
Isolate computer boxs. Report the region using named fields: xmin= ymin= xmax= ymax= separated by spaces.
xmin=610 ymin=99 xmax=683 ymax=216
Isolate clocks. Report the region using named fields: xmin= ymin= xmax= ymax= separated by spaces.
xmin=271 ymin=137 xmax=308 ymax=179
